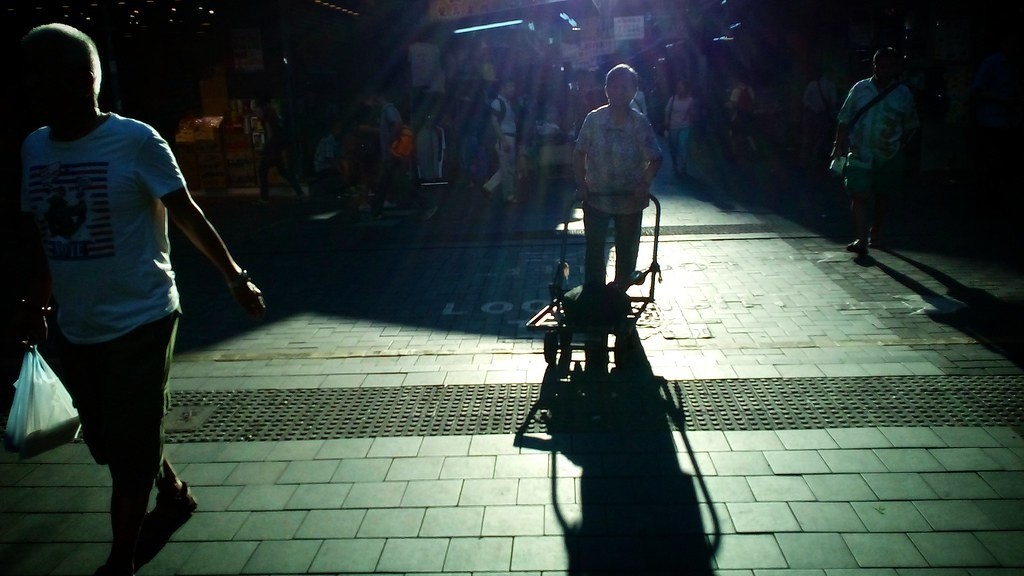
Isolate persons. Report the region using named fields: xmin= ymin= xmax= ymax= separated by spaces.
xmin=250 ymin=46 xmax=918 ymax=252
xmin=20 ymin=22 xmax=266 ymax=575
xmin=571 ymin=63 xmax=663 ymax=292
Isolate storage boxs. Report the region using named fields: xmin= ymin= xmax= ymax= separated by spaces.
xmin=175 ymin=71 xmax=291 ymax=190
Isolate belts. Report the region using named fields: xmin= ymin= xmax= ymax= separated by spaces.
xmin=502 ymin=131 xmax=518 ymax=137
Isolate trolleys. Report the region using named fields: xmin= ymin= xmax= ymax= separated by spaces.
xmin=527 ymin=191 xmax=660 ymax=362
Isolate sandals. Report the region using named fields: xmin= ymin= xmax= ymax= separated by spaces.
xmin=503 ymin=197 xmax=521 ymax=205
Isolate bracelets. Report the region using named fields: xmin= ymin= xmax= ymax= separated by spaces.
xmin=32 ymin=304 xmax=50 ymax=315
xmin=229 ymin=268 xmax=251 ymax=290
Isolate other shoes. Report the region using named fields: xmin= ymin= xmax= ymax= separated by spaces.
xmin=145 ymin=483 xmax=198 ymax=519
xmin=845 ymin=236 xmax=870 ymax=253
xmin=94 ymin=557 xmax=133 ymax=574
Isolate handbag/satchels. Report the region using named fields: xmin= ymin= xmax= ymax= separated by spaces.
xmin=3 ymin=345 xmax=81 ymax=459
xmin=390 ymin=123 xmax=414 ymax=161
